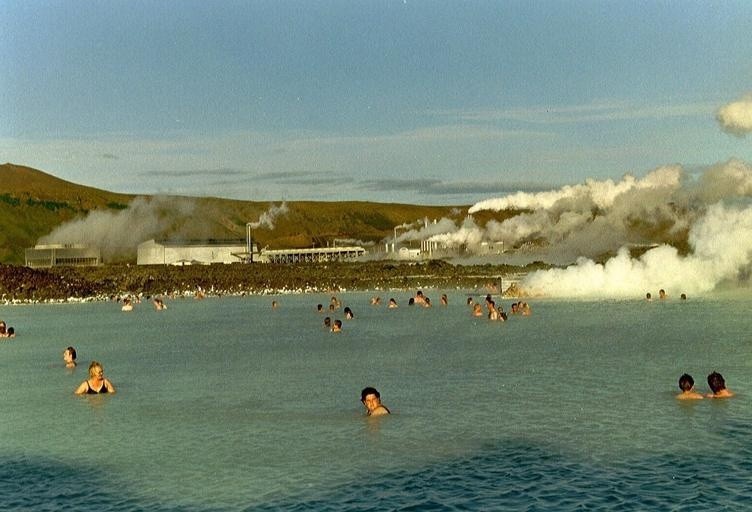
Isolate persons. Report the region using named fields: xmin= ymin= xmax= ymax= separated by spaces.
xmin=360 ymin=385 xmax=391 ymax=418
xmin=372 ymin=296 xmax=397 ymax=307
xmin=645 ymin=292 xmax=651 ymax=301
xmin=658 ymin=289 xmax=668 ymax=299
xmin=325 ymin=317 xmax=341 ymax=332
xmin=702 ymin=371 xmax=735 ymax=398
xmin=511 ymin=301 xmax=530 ymax=315
xmin=273 ymin=301 xmax=276 ymax=306
xmin=111 ymin=294 xmax=167 ymax=310
xmin=344 ymin=307 xmax=353 ymax=319
xmin=0 ymin=321 xmax=15 ymax=337
xmin=74 ymin=361 xmax=114 ymax=394
xmin=441 ymin=295 xmax=447 ymax=304
xmin=673 ymin=373 xmax=703 ymax=400
xmin=64 ymin=346 xmax=77 ymax=367
xmin=317 ymin=297 xmax=341 ymax=312
xmin=681 ymin=294 xmax=686 ymax=299
xmin=467 ymin=294 xmax=507 ymax=321
xmin=409 ymin=290 xmax=431 ymax=307
xmin=194 ymin=291 xmax=201 ymax=298
